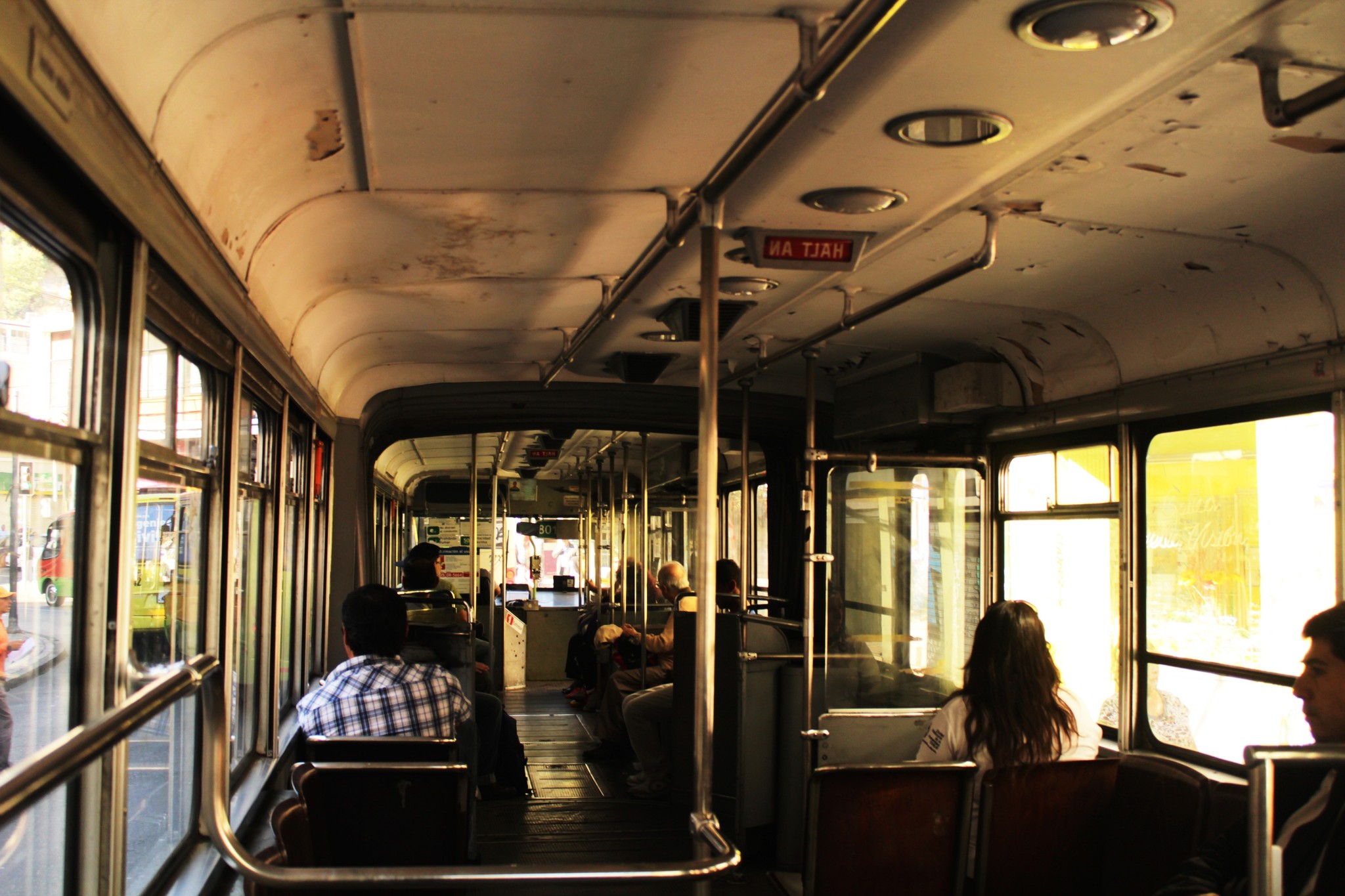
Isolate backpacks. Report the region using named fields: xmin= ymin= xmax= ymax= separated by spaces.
xmin=496 ymin=708 xmax=533 ymax=794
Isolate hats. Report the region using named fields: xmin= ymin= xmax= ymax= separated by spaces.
xmin=394 ymin=542 xmax=441 ymax=568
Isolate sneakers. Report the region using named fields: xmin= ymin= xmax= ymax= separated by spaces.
xmin=627 ymin=773 xmax=667 ymax=800
xmin=560 ymin=683 xmax=601 ymax=711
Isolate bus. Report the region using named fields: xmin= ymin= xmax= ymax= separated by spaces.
xmin=124 ymin=487 xmax=187 ymax=631
xmin=36 ymin=514 xmax=74 ymax=607
xmin=157 ymin=493 xmax=292 ymax=712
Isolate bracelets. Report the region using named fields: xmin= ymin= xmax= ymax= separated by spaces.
xmin=7 ymin=642 xmax=11 ymax=651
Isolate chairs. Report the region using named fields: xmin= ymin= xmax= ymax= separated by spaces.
xmin=267 ymin=542 xmax=1335 ymax=896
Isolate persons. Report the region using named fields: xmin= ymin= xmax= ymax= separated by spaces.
xmin=551 ymin=538 xmax=578 ymax=576
xmin=296 ymin=582 xmax=473 ymax=737
xmin=1099 ymin=638 xmax=1198 ymax=750
xmin=1199 ymin=600 xmax=1345 ymax=896
xmin=791 ymin=585 xmax=880 ymax=707
xmin=0 ymin=524 xmax=7 ymax=535
xmin=0 ymin=586 xmax=26 ymax=773
xmin=560 ymin=556 xmax=672 ymax=711
xmin=912 ymin=599 xmax=1103 ymax=881
xmin=627 ymin=558 xmax=756 ymax=799
xmin=582 ymin=560 xmax=696 ymax=761
xmin=396 ymin=542 xmax=529 ymax=799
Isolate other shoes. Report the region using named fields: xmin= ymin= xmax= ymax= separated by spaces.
xmin=582 ymin=743 xmax=608 ymax=760
xmin=482 ymin=785 xmax=516 ymax=800
